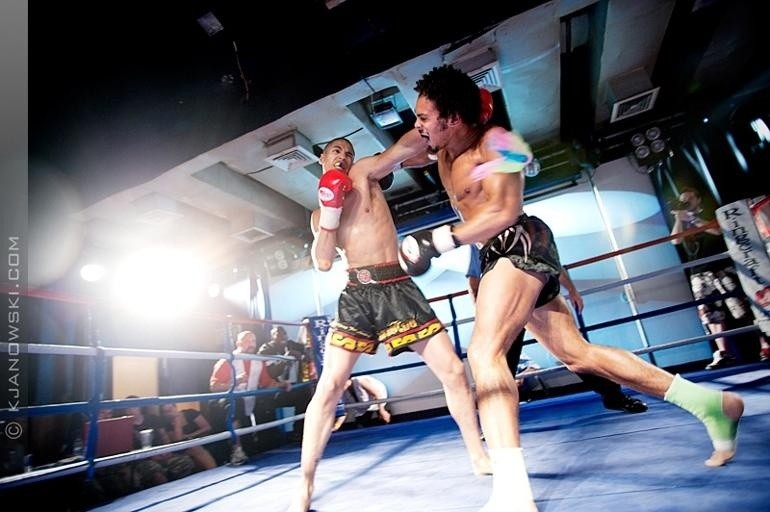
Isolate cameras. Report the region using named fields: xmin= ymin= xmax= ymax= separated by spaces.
xmin=668 ymin=198 xmax=690 ymax=213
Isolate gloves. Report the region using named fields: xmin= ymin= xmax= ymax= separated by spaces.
xmin=373 ymin=152 xmax=394 ymax=191
xmin=318 ymin=169 xmax=353 ymax=230
xmin=397 ymin=222 xmax=460 ymax=276
xmin=479 ymin=87 xmax=494 ymax=124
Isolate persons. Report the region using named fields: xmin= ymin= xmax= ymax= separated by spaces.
xmin=0 ymin=321 xmax=392 ymax=512
xmin=374 ymin=62 xmax=746 ymax=512
xmin=665 ymin=183 xmax=769 ymax=372
xmin=462 ymin=210 xmax=651 ymax=416
xmin=515 ymin=352 xmax=541 ymax=404
xmin=290 ymin=85 xmax=492 ymax=512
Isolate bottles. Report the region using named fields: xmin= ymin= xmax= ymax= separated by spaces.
xmin=73 ymin=438 xmax=83 ymax=456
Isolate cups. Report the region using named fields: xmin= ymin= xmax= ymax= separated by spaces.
xmin=140 ymin=429 xmax=154 ymax=449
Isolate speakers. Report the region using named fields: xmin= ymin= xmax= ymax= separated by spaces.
xmin=263 ymin=245 xmax=294 ymax=278
xmin=626 ymin=122 xmax=669 ymax=168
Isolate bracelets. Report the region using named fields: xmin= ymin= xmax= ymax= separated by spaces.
xmin=688 ymin=215 xmax=698 ymax=224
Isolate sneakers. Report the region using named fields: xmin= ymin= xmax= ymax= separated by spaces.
xmin=604 ymin=394 xmax=647 ymax=411
xmin=705 ymin=351 xmax=740 ymax=370
xmin=232 ymin=446 xmax=246 ymax=465
